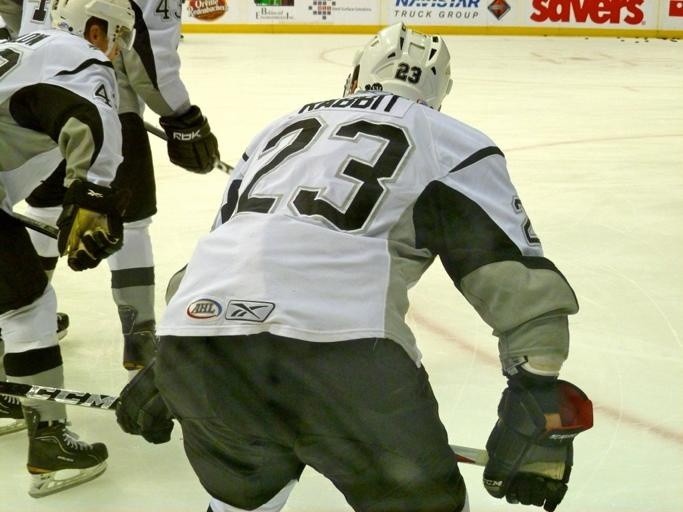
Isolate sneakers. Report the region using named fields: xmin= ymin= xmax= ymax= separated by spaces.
xmin=26 ymin=421 xmax=110 ymax=476
xmin=0 ymin=393 xmax=25 ymax=420
xmin=56 ymin=312 xmax=69 ymax=333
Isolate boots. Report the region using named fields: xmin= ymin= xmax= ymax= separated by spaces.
xmin=117 ymin=305 xmax=161 ymax=370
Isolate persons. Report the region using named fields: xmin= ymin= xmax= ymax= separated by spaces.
xmin=0 ymin=0 xmax=220 ymax=380
xmin=115 ymin=22 xmax=592 ymax=512
xmin=0 ymin=0 xmax=138 ymax=499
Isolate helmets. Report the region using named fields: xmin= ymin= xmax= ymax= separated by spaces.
xmin=50 ymin=0 xmax=138 ymax=51
xmin=351 ymin=22 xmax=451 ymax=110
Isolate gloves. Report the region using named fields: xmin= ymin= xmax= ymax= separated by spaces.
xmin=482 ymin=370 xmax=593 ymax=512
xmin=158 ymin=105 xmax=221 ymax=175
xmin=57 ymin=177 xmax=125 ymax=272
xmin=116 ymin=362 xmax=176 ymax=444
